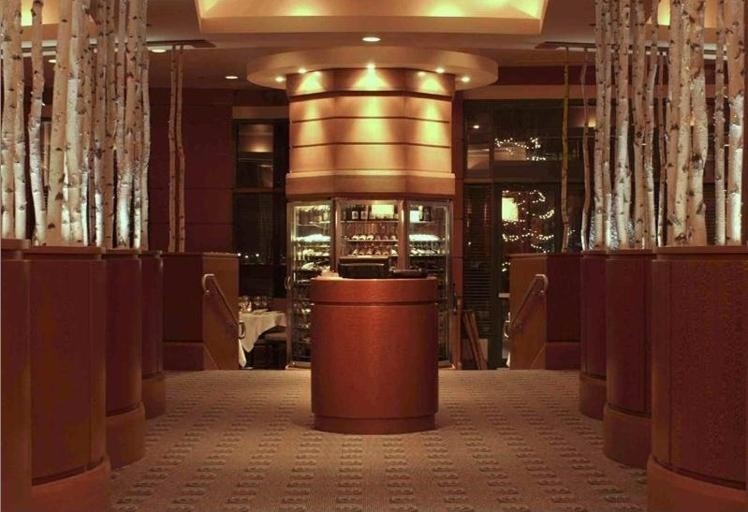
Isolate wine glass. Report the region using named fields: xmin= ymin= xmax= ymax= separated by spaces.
xmin=237 ymin=293 xmax=271 ymax=314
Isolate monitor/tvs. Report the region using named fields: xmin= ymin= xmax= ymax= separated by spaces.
xmin=336 ymin=254 xmax=389 ymax=279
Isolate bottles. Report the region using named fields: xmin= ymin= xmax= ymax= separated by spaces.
xmin=289 ymin=201 xmax=448 ymax=277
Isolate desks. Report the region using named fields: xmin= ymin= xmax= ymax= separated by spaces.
xmin=239 ymin=305 xmax=287 ymax=369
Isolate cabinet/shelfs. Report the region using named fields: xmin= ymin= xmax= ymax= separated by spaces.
xmin=287 ymin=198 xmax=452 ymax=368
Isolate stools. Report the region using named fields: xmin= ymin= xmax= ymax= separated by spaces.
xmin=264 ymin=328 xmax=288 ymax=369
